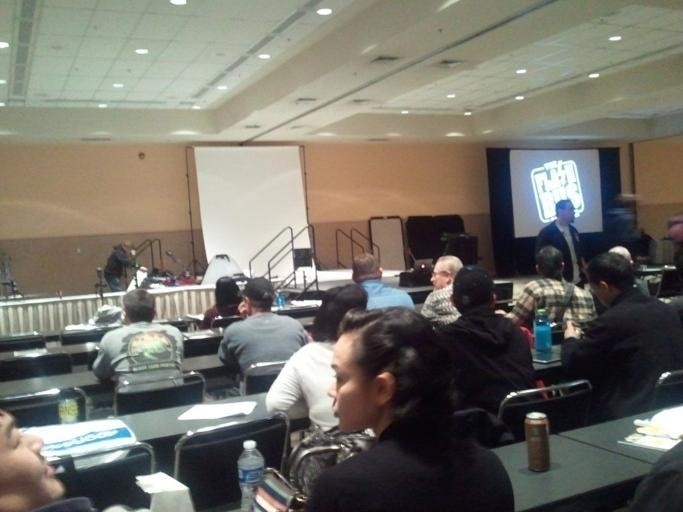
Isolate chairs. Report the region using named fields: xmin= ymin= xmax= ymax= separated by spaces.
xmin=0 ymin=258 xmax=682 ymax=512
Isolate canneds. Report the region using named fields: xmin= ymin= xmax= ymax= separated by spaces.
xmin=523 ymin=411 xmax=550 ymax=472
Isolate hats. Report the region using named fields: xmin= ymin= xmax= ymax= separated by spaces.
xmin=243 ymin=276 xmax=274 ymax=300
xmin=452 ymin=264 xmax=494 ymax=313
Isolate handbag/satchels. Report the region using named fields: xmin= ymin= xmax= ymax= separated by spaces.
xmin=286 ymin=427 xmax=378 ymax=498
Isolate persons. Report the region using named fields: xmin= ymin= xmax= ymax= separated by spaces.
xmin=103 ymin=239 xmax=148 ymax=292
xmin=421 ymin=246 xmax=683 ymax=428
xmin=266 ymin=285 xmax=375 ymax=494
xmin=304 ymin=306 xmax=514 ymax=512
xmin=93 ymin=289 xmax=184 ymax=415
xmin=600 ymin=194 xmax=643 ymax=255
xmin=0 ymin=407 xmax=98 ymax=512
xmin=534 ymin=200 xmax=589 ymax=288
xmin=202 ymin=276 xmax=313 ymax=399
xmin=668 ymin=214 xmax=683 ymax=277
xmin=353 ymin=252 xmax=416 ymax=311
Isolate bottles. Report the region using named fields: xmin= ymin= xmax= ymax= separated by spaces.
xmin=54 ymin=385 xmax=83 ymax=424
xmin=276 ymin=292 xmax=284 ymax=308
xmin=533 ymin=308 xmax=553 ymax=361
xmin=234 ymin=439 xmax=263 ymax=511
